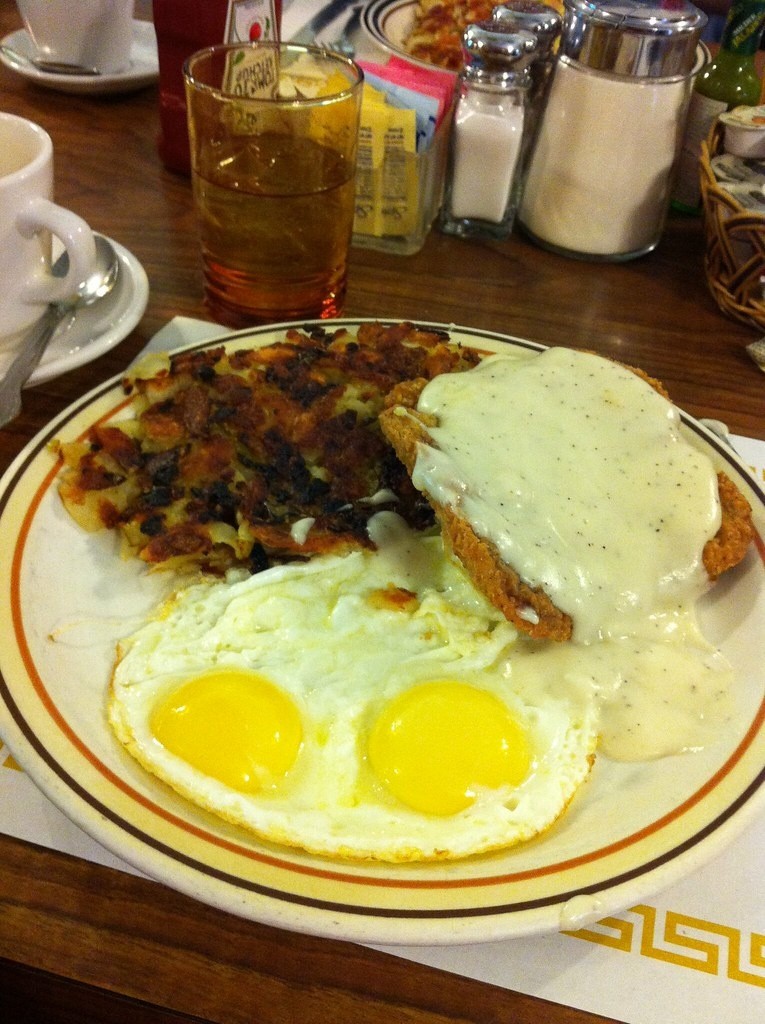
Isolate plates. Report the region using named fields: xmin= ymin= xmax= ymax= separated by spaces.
xmin=0 ymin=318 xmax=765 ymax=946
xmin=359 ymin=0 xmax=713 ymax=93
xmin=0 ymin=230 xmax=151 ymax=390
xmin=0 ymin=19 xmax=160 ymax=95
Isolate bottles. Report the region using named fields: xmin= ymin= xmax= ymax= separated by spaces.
xmin=151 ymin=0 xmax=283 ymax=176
xmin=434 ymin=20 xmax=539 ymax=243
xmin=670 ymin=0 xmax=765 ymax=214
xmin=492 ymin=2 xmax=564 ymax=102
xmin=516 ymin=0 xmax=709 ymax=264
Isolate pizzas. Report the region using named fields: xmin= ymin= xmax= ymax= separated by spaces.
xmin=404 ymin=0 xmax=565 ymax=75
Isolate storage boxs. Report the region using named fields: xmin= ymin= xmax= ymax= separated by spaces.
xmin=350 ymin=98 xmax=455 ymax=257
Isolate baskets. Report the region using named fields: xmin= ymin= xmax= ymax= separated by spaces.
xmin=695 ymin=98 xmax=765 ymax=335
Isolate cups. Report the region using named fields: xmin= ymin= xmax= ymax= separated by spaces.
xmin=718 ymin=105 xmax=765 ymax=159
xmin=0 ymin=111 xmax=96 ymax=354
xmin=181 ymin=40 xmax=365 ymax=330
xmin=15 ymin=0 xmax=135 ymax=75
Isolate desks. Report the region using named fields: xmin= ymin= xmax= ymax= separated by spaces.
xmin=0 ymin=0 xmax=763 ymax=1024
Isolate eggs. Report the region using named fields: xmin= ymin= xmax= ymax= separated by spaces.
xmin=109 ymin=537 xmax=595 ymax=863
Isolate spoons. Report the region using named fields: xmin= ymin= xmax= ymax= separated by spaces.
xmin=0 ymin=45 xmax=102 ymax=76
xmin=0 ymin=235 xmax=119 ymax=429
